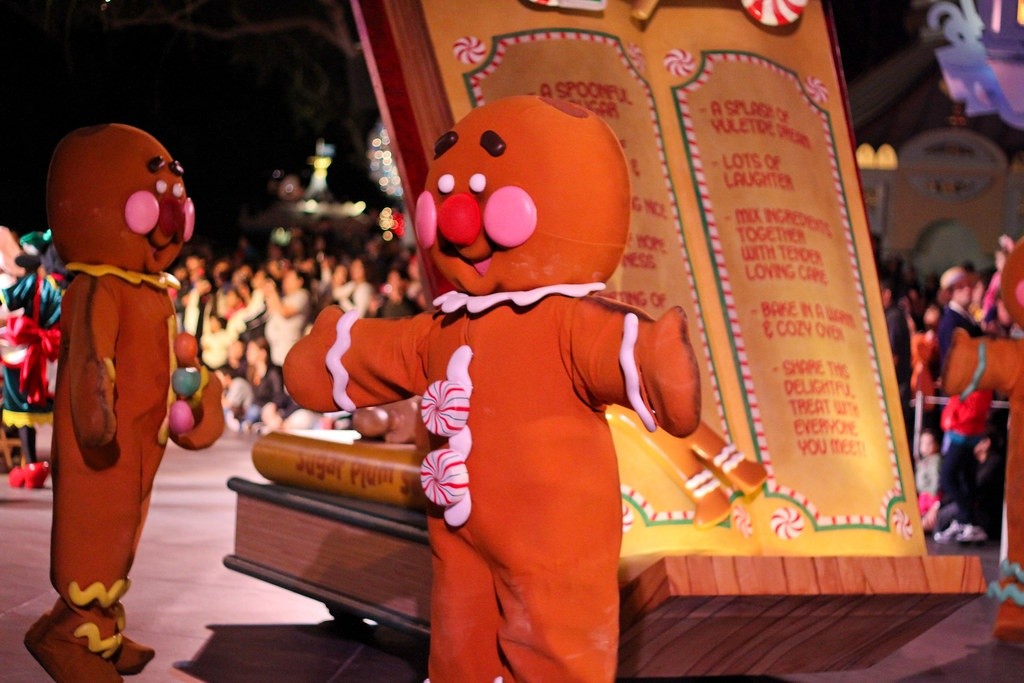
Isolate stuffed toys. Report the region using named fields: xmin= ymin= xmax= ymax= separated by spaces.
xmin=0 ymin=226 xmax=62 ymax=489
xmin=945 ymin=238 xmax=1024 ymax=648
xmin=23 ymin=123 xmax=224 ymax=683
xmin=282 ymin=97 xmax=702 ymax=683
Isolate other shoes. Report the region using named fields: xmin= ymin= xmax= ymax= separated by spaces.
xmin=934 ymin=520 xmax=965 ymax=543
xmin=956 ymin=524 xmax=988 ymax=541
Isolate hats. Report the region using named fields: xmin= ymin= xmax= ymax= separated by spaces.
xmin=937 ymin=265 xmax=980 ymax=304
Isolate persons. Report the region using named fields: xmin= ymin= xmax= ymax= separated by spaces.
xmin=870 ymin=233 xmax=1024 ymax=542
xmin=167 ymin=251 xmax=432 ymax=436
